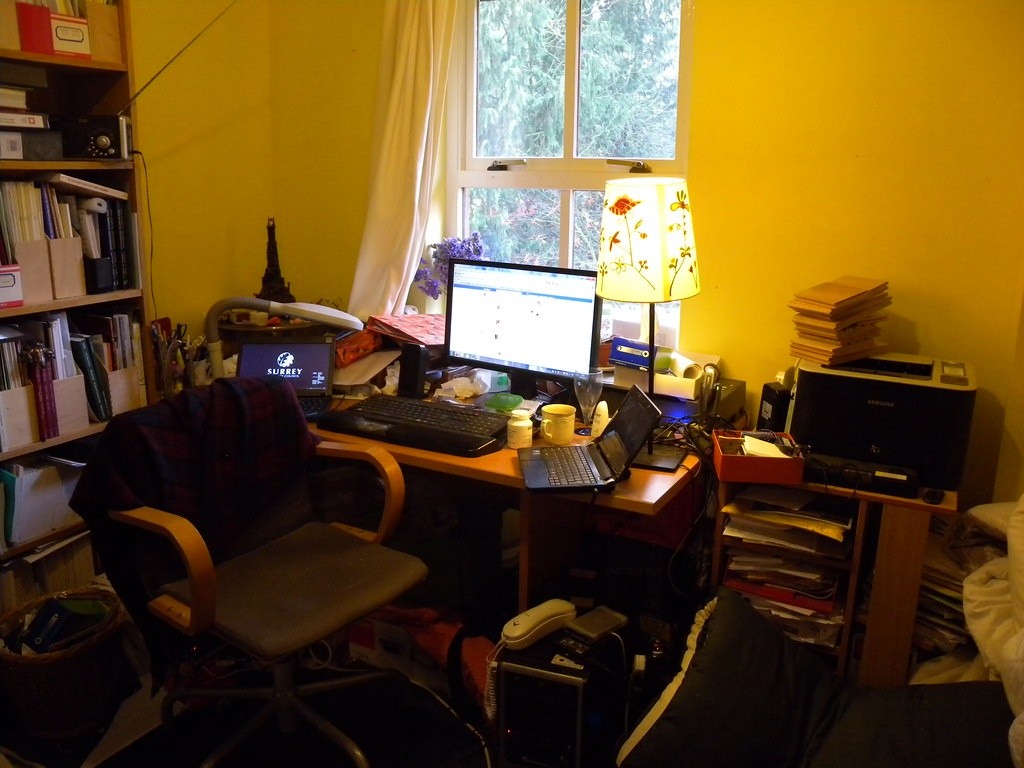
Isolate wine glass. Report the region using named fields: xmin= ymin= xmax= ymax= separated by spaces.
xmin=573 ymin=368 xmax=603 ymax=436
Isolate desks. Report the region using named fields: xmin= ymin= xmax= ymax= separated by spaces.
xmin=302 ymin=387 xmax=706 ymax=613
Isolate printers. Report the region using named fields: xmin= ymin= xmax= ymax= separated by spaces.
xmin=785 ymin=347 xmax=978 ymax=493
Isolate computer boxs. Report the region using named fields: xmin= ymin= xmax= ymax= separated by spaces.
xmin=496 ymin=627 xmax=637 ymax=768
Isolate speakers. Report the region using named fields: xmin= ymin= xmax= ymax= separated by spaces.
xmin=398 ymin=342 xmax=429 ymax=399
xmin=756 ymin=382 xmax=790 ymax=433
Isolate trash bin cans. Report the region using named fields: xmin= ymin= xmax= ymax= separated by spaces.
xmin=0 ymin=590 xmax=128 ymax=739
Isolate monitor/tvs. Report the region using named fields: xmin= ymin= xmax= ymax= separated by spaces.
xmin=444 ymin=259 xmax=597 ymax=404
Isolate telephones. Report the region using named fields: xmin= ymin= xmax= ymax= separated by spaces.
xmin=501 ymin=598 xmax=577 ymax=650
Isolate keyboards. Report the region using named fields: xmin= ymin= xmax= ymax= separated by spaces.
xmin=317 ymin=393 xmax=511 ymax=457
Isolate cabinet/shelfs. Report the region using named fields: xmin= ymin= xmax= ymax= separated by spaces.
xmin=0 ymin=0 xmax=160 ymax=640
xmin=713 ymin=481 xmax=960 ymax=692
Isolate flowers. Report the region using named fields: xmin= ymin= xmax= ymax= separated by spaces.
xmin=412 ymin=230 xmax=484 ymax=301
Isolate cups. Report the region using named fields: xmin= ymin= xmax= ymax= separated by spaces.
xmin=540 ymin=404 xmax=576 ymax=446
xmin=189 ymin=358 xmax=212 ymax=387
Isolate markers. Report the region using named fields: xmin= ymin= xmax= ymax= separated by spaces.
xmin=176 ymin=348 xmax=185 ymax=369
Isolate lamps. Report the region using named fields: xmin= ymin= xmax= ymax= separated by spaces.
xmin=204 ymin=297 xmax=363 ymax=380
xmin=595 ymin=167 xmax=699 ymax=473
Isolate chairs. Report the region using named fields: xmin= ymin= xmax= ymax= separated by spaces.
xmin=612 ymin=589 xmax=1016 ymax=768
xmin=67 ymin=374 xmax=430 ymax=768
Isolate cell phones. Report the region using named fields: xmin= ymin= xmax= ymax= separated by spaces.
xmin=924 ymin=490 xmax=945 ymax=504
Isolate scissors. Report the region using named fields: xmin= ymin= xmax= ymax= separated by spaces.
xmin=169 ymin=324 xmax=187 ymax=341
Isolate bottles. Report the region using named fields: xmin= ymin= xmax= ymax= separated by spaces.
xmin=506 ymin=410 xmax=533 ymax=449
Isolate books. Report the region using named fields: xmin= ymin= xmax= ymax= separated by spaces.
xmin=1 ymin=168 xmax=152 ymax=608
xmin=714 ymin=488 xmax=989 ymax=662
xmin=789 ymin=273 xmax=893 ymax=365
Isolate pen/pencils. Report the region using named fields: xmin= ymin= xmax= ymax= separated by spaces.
xmin=186 ymin=334 xmax=190 ymax=347
xmin=185 ymin=354 xmax=190 ymax=370
xmin=152 ymin=325 xmax=158 ymax=336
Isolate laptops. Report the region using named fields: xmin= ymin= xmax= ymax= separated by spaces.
xmin=517 ymin=383 xmax=662 ymax=490
xmin=235 ymin=336 xmax=336 ymax=420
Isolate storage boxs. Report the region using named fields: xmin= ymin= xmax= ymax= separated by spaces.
xmin=0 ymin=378 xmax=41 ymax=450
xmin=15 ymin=2 xmax=55 ymax=56
xmin=86 ymin=2 xmax=122 ymax=64
xmin=0 ymin=256 xmax=24 ymax=309
xmin=93 ymin=352 xmax=141 ymax=417
xmin=82 ymin=252 xmax=113 ymax=295
xmin=710 ymin=429 xmax=806 ymax=484
xmin=53 ymin=360 xmax=90 ymax=435
xmin=9 ymin=238 xmax=54 ymax=305
xmin=50 ymin=12 xmax=91 ymax=61
xmin=44 ymin=227 xmax=86 ymax=298
